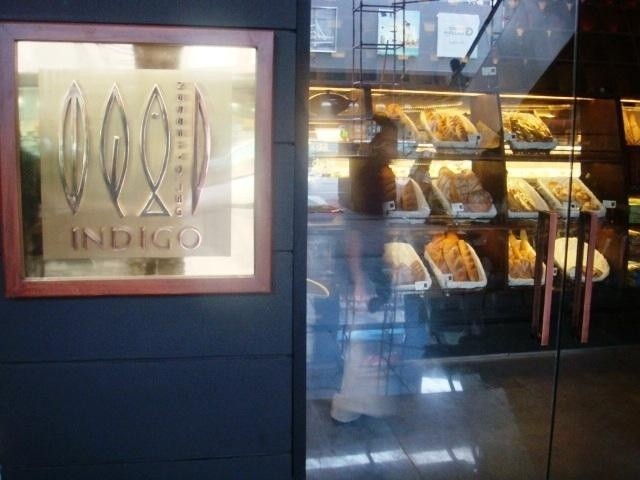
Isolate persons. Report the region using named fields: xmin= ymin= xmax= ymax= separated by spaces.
xmin=330 ymin=116 xmax=399 ymax=427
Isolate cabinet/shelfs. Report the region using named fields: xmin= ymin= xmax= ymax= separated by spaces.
xmin=307 ymin=85 xmax=640 ymax=327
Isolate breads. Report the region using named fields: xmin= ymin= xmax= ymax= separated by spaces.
xmin=437 ymin=165 xmax=493 ymax=212
xmin=549 ymin=178 xmax=599 ymax=210
xmin=402 ymin=180 xmax=423 ymax=212
xmin=424 ymin=109 xmax=472 ymax=142
xmin=623 ymin=109 xmax=640 ymax=145
xmin=508 ymin=185 xmax=537 ymax=213
xmin=499 ymin=110 xmax=555 ymax=143
xmin=426 ymin=232 xmax=478 ymax=284
xmin=506 ymin=229 xmax=541 ymax=282
